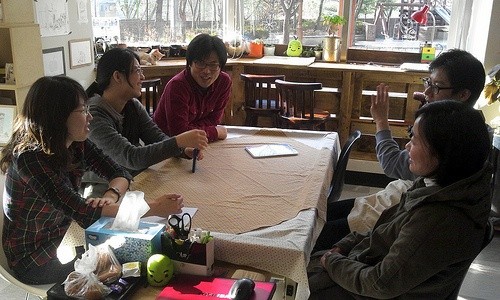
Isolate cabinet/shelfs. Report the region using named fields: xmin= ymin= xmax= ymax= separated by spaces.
xmin=0 ymin=0 xmax=42 ymax=149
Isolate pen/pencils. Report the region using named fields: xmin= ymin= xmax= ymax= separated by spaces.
xmin=192 ymin=149 xmax=199 ymax=173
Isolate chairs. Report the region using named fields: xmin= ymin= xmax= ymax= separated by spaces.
xmin=136 ymin=76 xmax=163 ymax=115
xmin=275 ymin=80 xmax=329 ymax=132
xmin=0 ymin=176 xmax=88 ymax=300
xmin=328 ymin=128 xmax=364 ymax=203
xmin=240 ymin=74 xmax=286 ymax=126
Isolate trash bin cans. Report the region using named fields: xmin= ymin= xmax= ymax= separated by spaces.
xmin=366 ymin=24 xmax=377 ymax=41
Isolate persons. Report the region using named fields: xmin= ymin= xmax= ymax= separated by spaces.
xmin=311 ymin=47 xmax=486 ymax=254
xmin=0 ymin=75 xmax=184 ymax=285
xmin=153 ymin=34 xmax=233 ymax=140
xmin=83 ymin=47 xmax=208 ymax=199
xmin=306 ymin=100 xmax=494 ymax=300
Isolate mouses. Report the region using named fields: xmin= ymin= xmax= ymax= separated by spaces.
xmin=228 ymin=278 xmax=256 ymax=300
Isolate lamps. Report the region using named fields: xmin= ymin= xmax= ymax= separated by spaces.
xmin=410 ymin=5 xmax=435 ymax=65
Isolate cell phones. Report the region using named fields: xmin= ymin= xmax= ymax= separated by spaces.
xmin=192 ymin=148 xmax=197 ymax=173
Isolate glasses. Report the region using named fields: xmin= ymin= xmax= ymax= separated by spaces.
xmin=194 ymin=60 xmax=220 ymax=71
xmin=423 ymin=77 xmax=454 ymax=94
xmin=72 ymin=108 xmax=90 ymax=114
xmin=135 ymin=68 xmax=143 ymax=75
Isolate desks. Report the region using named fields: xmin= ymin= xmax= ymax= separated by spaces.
xmin=113 ymin=125 xmax=339 ymax=300
xmin=138 ymin=53 xmax=432 ymax=149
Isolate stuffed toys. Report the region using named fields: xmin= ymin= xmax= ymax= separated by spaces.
xmin=283 ymin=36 xmax=302 ymax=57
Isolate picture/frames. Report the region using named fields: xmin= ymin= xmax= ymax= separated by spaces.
xmin=0 ymin=104 xmax=17 ymax=146
xmin=68 ymin=39 xmax=95 ymax=68
xmin=42 ymin=47 xmax=66 ymax=76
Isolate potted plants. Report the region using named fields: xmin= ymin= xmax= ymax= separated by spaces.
xmin=249 ymin=38 xmax=264 ymax=58
xmin=264 ymin=41 xmax=276 ymax=56
xmin=314 ymin=40 xmax=323 ymax=60
xmin=321 ymin=13 xmax=347 ymax=63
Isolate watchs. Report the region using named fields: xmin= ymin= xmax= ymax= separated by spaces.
xmin=105 ymin=187 xmax=121 ymax=204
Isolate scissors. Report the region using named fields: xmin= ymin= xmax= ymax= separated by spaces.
xmin=168 ymin=213 xmax=191 ymax=240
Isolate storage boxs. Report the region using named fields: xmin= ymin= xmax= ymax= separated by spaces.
xmin=161 ymin=232 xmax=216 ymax=279
